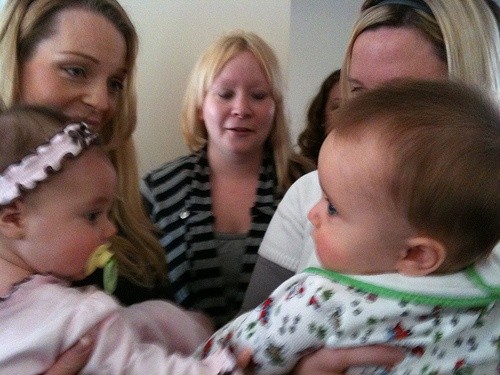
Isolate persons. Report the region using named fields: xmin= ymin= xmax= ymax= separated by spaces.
xmin=0 ymin=0 xmax=165 ymax=375
xmin=196 ymin=74 xmax=499 ymax=375
xmin=1 ymin=106 xmax=211 ymax=375
xmin=139 ymin=30 xmax=319 ymax=331
xmin=298 ymin=69 xmax=343 ymax=169
xmin=242 ymin=0 xmax=500 ymax=375
